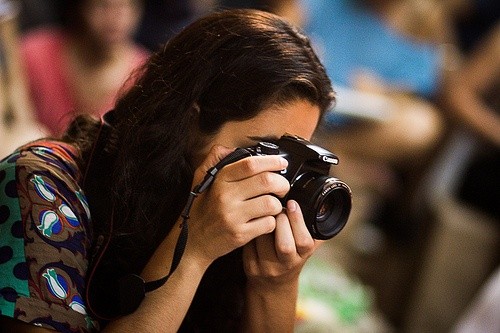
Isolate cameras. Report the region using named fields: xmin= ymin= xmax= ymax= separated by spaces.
xmin=234 ymin=133 xmax=352 ymax=240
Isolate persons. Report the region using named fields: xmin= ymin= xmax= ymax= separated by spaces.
xmin=0 ymin=8 xmax=336 ymax=333
xmin=0 ymin=0 xmax=500 ymax=333
